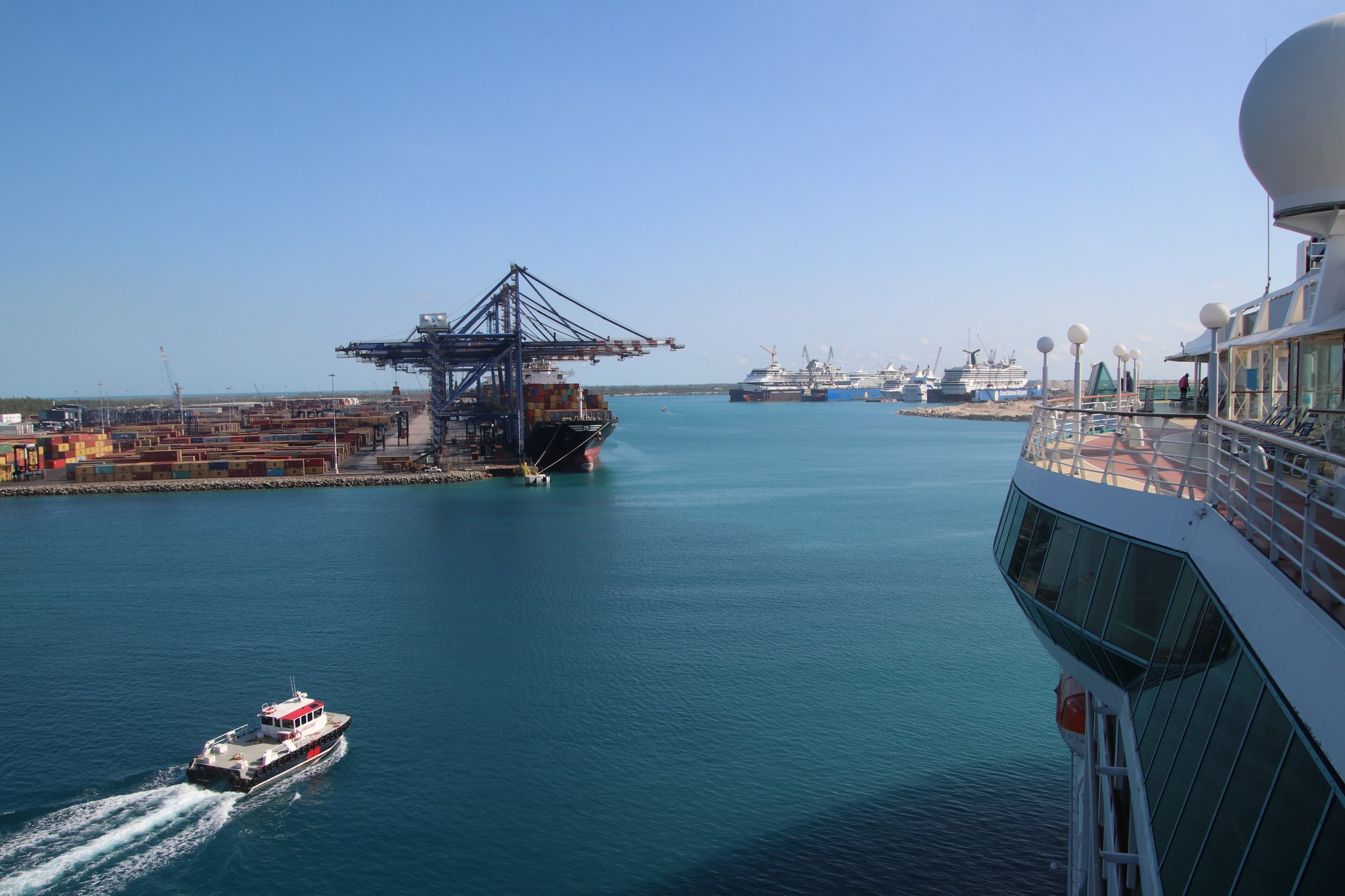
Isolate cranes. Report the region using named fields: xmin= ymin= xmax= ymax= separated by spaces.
xmin=334 ymin=264 xmax=686 ymax=460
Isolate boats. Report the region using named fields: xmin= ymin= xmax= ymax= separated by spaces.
xmin=879 ymin=365 xmax=914 ymax=401
xmin=940 ymin=328 xmax=1028 ymax=395
xmin=902 ymin=346 xmax=942 ymax=403
xmin=991 ymin=13 xmax=1345 ymax=896
xmin=728 ymin=344 xmax=886 ymax=404
xmin=185 ymin=675 xmax=353 ymax=800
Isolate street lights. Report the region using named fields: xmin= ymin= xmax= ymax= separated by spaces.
xmin=1113 ymin=344 xmax=1126 ymax=436
xmin=1199 ymin=301 xmax=1230 ymax=510
xmin=1067 ymin=324 xmax=1089 ymax=476
xmin=1037 ymin=337 xmax=1054 ymax=423
xmin=97 ymin=382 xmax=110 ymax=434
xmin=1129 ymin=348 xmax=1144 ymax=447
xmin=375 ymin=382 xmax=389 ymax=412
xmin=1121 ymin=353 xmax=1130 ymax=416
xmin=227 ymin=387 xmax=235 ymax=423
xmin=284 ymin=385 xmax=287 ymax=410
xmin=126 ymin=390 xmax=130 ymax=408
xmin=1135 ymin=362 xmax=1142 ymax=410
xmin=75 ymin=391 xmax=78 ymax=405
xmin=329 ymin=373 xmax=339 ymax=474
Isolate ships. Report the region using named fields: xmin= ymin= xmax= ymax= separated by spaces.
xmin=480 ymin=359 xmax=618 ymax=473
